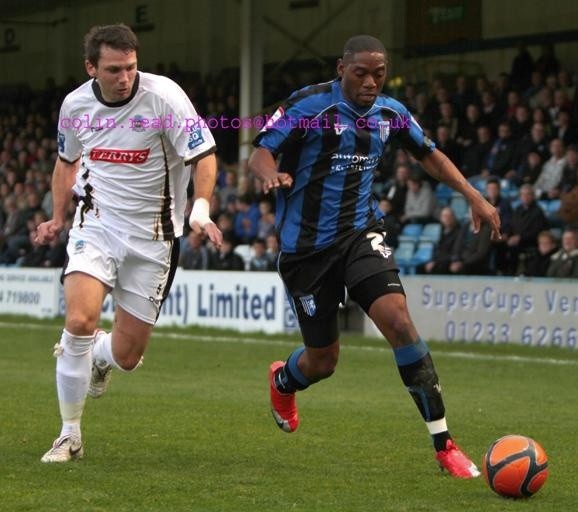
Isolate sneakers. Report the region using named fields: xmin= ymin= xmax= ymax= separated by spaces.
xmin=435 ymin=439 xmax=481 ymax=480
xmin=41 ymin=434 xmax=84 ymax=464
xmin=87 ymin=329 xmax=110 ymax=399
xmin=270 ymin=361 xmax=298 ymax=433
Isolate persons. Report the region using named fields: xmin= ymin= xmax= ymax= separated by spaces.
xmin=1 ymin=42 xmax=578 ymax=277
xmin=247 ymin=34 xmax=503 ymax=480
xmin=33 ymin=22 xmax=225 ymax=465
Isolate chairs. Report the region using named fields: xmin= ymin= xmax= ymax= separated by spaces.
xmin=392 ymin=175 xmax=562 ymax=275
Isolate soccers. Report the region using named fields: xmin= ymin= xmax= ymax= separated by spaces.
xmin=484 ymin=435 xmax=548 ymax=499
